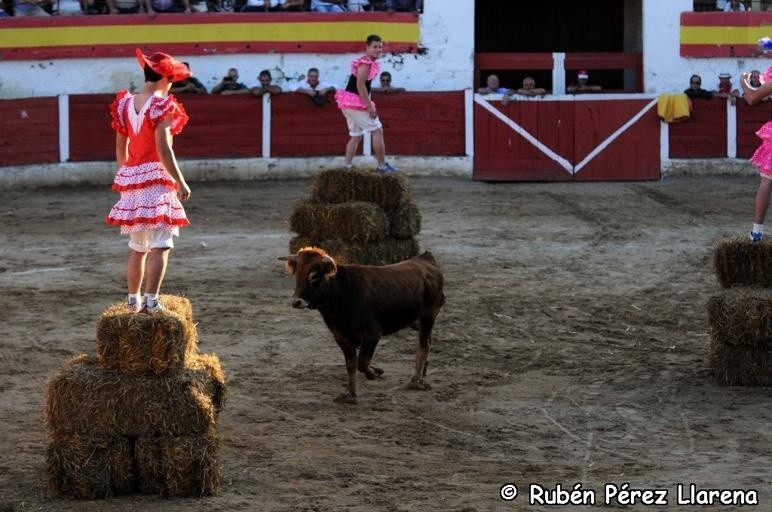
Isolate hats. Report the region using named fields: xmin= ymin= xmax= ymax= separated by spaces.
xmin=135 ymin=47 xmax=193 ymax=83
xmin=719 ymin=72 xmax=731 ymax=79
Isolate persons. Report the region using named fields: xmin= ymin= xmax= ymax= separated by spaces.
xmin=332 ymin=34 xmax=397 ymax=175
xmin=516 ymin=77 xmax=545 ymax=97
xmin=714 ymin=0 xmax=752 ymax=13
xmin=477 ymin=75 xmax=515 ymax=107
xmin=210 ymin=68 xmax=250 ymax=96
xmin=738 ymin=72 xmax=772 ymax=243
xmin=566 ymin=68 xmax=601 ymax=93
xmin=108 ymin=49 xmax=190 ymax=316
xmin=170 ymin=62 xmax=208 ymax=94
xmin=0 ymin=0 xmax=421 ymax=19
xmin=368 ymin=72 xmax=405 ymax=94
xmin=250 ymin=71 xmax=282 ymax=96
xmin=287 ymin=68 xmax=339 ymax=106
xmin=682 ymin=70 xmax=771 ymax=106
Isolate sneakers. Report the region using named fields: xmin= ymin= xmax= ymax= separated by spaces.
xmin=143 ymin=303 xmax=166 ymax=315
xmin=749 ymin=231 xmax=763 ymax=242
xmin=127 ymin=302 xmax=141 ymax=313
xmin=376 ymin=162 xmax=399 ymax=173
xmin=343 ymin=164 xmax=353 ymax=170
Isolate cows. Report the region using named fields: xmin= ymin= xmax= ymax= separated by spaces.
xmin=276 ymin=245 xmax=445 ymax=404
